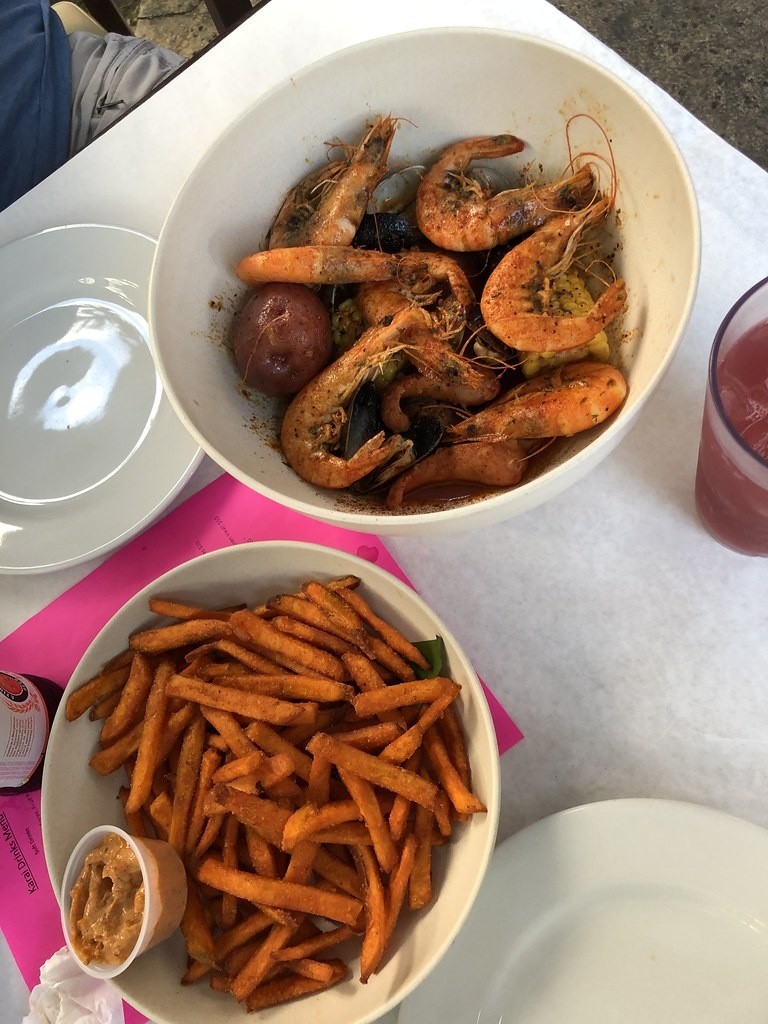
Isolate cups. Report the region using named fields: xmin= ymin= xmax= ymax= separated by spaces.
xmin=694 ymin=274 xmax=768 ymax=559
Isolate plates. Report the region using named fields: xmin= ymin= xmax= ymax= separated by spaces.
xmin=0 ymin=223 xmax=208 ymax=574
xmin=397 ymin=798 xmax=768 ymax=1024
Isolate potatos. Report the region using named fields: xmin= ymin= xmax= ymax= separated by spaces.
xmin=232 ymin=283 xmax=332 ymax=395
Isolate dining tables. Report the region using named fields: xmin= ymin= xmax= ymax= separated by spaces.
xmin=0 ymin=0 xmax=768 ymax=1024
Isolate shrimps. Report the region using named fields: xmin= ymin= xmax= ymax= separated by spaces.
xmin=227 ymin=109 xmax=629 ymax=512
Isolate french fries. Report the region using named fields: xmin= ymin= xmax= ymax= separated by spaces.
xmin=65 ymin=574 xmax=488 ymax=1013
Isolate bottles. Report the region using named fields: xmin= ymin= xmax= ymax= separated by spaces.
xmin=0 ymin=671 xmax=66 ymax=795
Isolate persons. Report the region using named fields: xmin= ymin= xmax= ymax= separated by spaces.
xmin=1 ymin=3 xmax=194 ymax=208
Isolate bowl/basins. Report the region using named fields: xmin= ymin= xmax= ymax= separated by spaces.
xmin=60 ymin=825 xmax=187 ymax=978
xmin=147 ymin=26 xmax=700 ymax=535
xmin=39 ymin=541 xmax=500 ymax=1024
